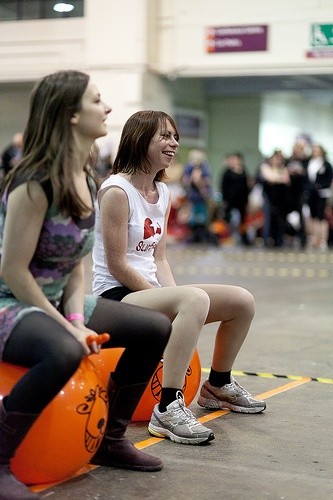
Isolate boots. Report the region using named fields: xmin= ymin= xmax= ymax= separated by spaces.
xmin=89 ymin=371 xmax=164 ymax=472
xmin=0 ymin=395 xmax=41 ymax=500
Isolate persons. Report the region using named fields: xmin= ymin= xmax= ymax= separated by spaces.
xmin=95 ymin=151 xmax=112 ymax=189
xmin=178 ymin=136 xmax=333 ymax=250
xmin=2 ymin=132 xmax=24 ymax=177
xmin=0 ymin=71 xmax=171 ymax=500
xmin=92 ymin=111 xmax=266 ymax=445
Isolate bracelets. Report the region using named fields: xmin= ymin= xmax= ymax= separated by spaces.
xmin=65 ymin=312 xmax=85 ymax=322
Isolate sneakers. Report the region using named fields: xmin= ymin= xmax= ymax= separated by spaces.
xmin=147 ymin=390 xmax=215 ymax=445
xmin=197 ymin=374 xmax=266 ymax=414
